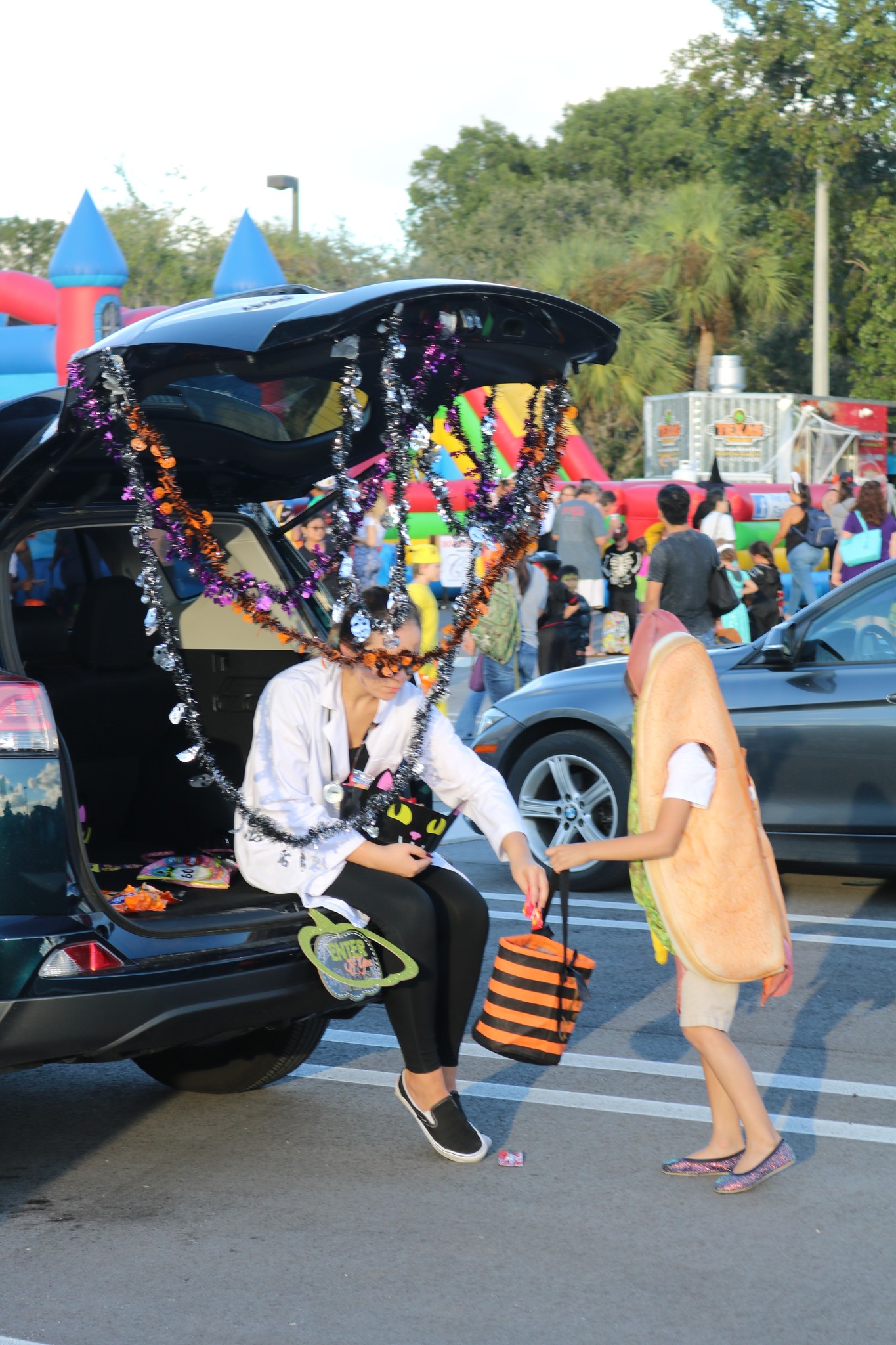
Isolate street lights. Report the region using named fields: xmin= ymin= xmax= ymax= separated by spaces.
xmin=267 ymin=173 xmax=302 ymax=286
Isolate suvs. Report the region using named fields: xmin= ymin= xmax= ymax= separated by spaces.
xmin=0 ymin=278 xmax=627 ymax=1101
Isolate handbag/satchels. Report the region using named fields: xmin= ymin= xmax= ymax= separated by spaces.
xmin=705 ymin=534 xmax=740 ymax=618
xmin=473 ymin=866 xmax=595 ymax=1065
xmin=838 ymin=510 xmax=883 ymax=566
xmin=471 ymin=653 xmax=484 ymax=692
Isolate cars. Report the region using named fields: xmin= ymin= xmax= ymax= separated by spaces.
xmin=464 ymin=558 xmax=896 ymax=895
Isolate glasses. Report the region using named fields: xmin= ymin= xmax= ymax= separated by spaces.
xmin=307 ymin=526 xmax=326 ymax=532
xmin=398 ymin=664 xmax=416 ymax=675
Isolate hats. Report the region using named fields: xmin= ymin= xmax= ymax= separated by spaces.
xmin=561 ymin=563 xmax=577 ymax=578
xmin=697 ymin=458 xmax=734 ymax=489
xmin=840 ymin=472 xmax=858 ymax=487
xmin=612 ymin=524 xmax=627 ymax=540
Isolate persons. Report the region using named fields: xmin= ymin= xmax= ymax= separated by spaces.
xmin=542 ymin=609 xmax=797 ymax=1194
xmin=233 ymin=588 xmax=552 ymax=1161
xmin=270 ymin=476 xmax=450 ymax=716
xmin=455 ymin=475 xmax=894 ymax=744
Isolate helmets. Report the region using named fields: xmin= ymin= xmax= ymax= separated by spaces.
xmin=528 ymin=551 xmax=560 ymax=573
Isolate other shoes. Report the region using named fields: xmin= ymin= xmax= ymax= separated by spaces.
xmin=394 ymin=1068 xmax=493 ymax=1163
xmin=661 ymin=1146 xmax=748 ymax=1176
xmin=712 ymin=1136 xmax=798 ymax=1195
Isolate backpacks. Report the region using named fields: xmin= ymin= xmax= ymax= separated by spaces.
xmin=791 ymin=502 xmax=835 ymax=546
